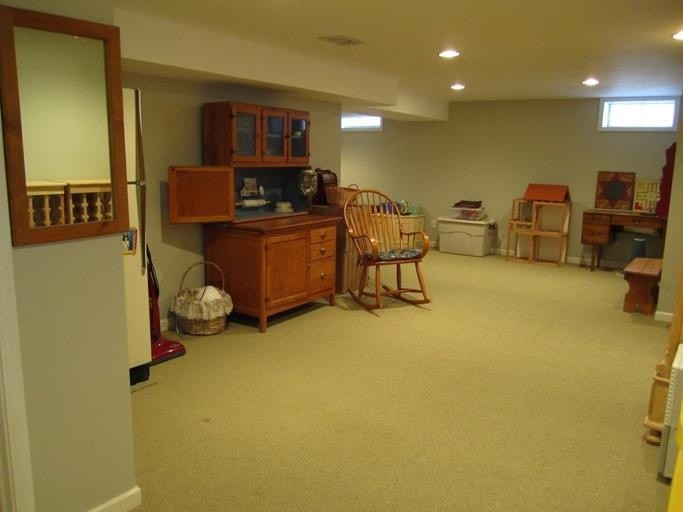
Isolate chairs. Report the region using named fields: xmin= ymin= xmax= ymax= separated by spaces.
xmin=343 ymin=189 xmax=431 ymax=308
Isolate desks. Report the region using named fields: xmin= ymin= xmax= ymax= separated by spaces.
xmin=580 ymin=208 xmax=668 ymax=272
xmin=371 ymin=212 xmax=424 ymax=248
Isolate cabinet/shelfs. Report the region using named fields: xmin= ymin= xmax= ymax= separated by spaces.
xmin=167 ymin=100 xmax=344 ymax=332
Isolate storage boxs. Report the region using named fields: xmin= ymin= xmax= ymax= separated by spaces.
xmin=309 ymin=205 xmax=372 ymax=294
xmin=437 ymin=216 xmax=496 ymax=258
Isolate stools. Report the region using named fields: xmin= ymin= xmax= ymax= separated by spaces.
xmin=622 ymin=257 xmax=663 ymax=315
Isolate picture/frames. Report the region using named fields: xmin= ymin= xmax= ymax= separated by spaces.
xmin=594 ymin=171 xmax=635 ymax=213
xmin=633 ymin=180 xmax=661 ymax=215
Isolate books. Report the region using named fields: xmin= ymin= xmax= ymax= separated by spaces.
xmin=452 ymin=200 xmax=482 ymax=209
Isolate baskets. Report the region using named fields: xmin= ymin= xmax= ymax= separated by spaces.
xmin=170 ymin=261 xmax=233 ymax=336
xmin=326 ymin=183 xmax=360 ymax=206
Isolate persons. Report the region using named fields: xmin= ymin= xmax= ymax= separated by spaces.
xmin=123 ymin=236 xmax=129 ymax=250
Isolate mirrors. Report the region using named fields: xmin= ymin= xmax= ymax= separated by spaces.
xmin=0 ymin=4 xmax=131 ymax=247
